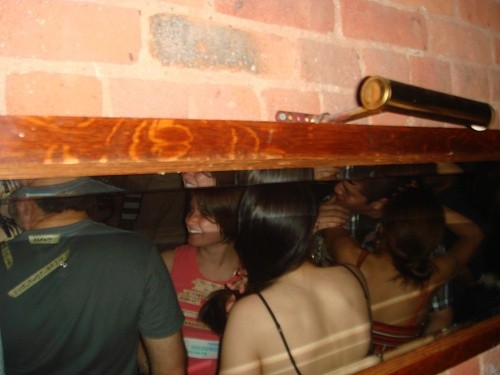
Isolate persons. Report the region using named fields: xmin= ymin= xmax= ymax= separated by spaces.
xmin=181 ymin=173 xmax=247 ymax=189
xmin=200 ymin=182 xmax=372 ymax=375
xmin=242 ymin=169 xmax=314 ymax=185
xmin=311 ymin=166 xmax=436 ymax=184
xmin=307 ymin=177 xmax=454 ymax=345
xmin=319 ymin=184 xmax=485 ymax=357
xmin=10 ymin=178 xmax=129 ymax=194
xmin=0 ymin=195 xmax=188 ymax=375
xmin=156 ymin=186 xmax=249 ymax=375
